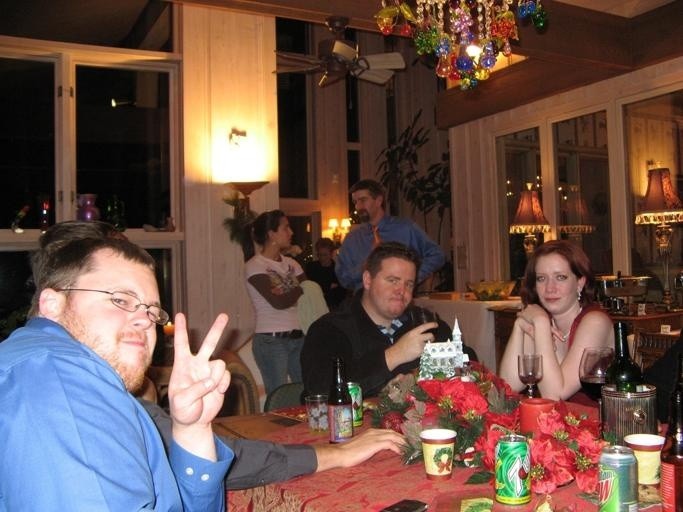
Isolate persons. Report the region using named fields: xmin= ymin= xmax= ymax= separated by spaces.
xmin=32 ymin=220 xmax=407 ymax=491
xmin=643 ymin=331 xmax=683 ymax=433
xmin=499 ymin=239 xmax=615 ymax=401
xmin=1 ymin=237 xmax=233 ymax=512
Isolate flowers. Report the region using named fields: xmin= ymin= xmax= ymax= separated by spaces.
xmin=375 ymin=372 xmax=601 ymax=492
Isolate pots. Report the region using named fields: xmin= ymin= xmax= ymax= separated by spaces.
xmin=594 ymin=274 xmax=652 ymax=317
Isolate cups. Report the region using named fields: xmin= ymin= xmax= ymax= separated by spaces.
xmin=304 ymin=393 xmax=329 ymax=431
xmin=623 ymin=434 xmax=667 ymax=505
xmin=418 ymin=427 xmax=457 ymax=482
xmin=408 ymin=304 xmax=439 ymax=336
xmin=518 ymin=399 xmax=556 ymax=439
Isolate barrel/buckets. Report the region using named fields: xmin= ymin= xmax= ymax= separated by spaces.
xmin=600 ymin=384 xmax=655 ymax=443
xmin=619 ymin=276 xmax=653 ymax=303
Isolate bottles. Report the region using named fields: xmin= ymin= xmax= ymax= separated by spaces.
xmin=609 ymin=319 xmax=643 ymax=392
xmin=326 ymin=358 xmax=353 ymax=444
xmin=104 ymin=194 xmax=125 ymax=232
xmin=660 ymin=391 xmax=682 ymax=511
xmin=79 ymin=195 xmax=101 ymax=223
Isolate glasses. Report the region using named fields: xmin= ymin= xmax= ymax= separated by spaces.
xmin=55 ymin=288 xmax=169 ymax=326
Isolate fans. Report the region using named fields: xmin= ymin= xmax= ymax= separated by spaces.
xmin=274 ymin=11 xmax=405 ymax=91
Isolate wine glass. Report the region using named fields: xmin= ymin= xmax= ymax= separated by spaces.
xmin=517 ymin=354 xmax=543 ymax=397
xmin=578 ymin=345 xmax=614 ymax=433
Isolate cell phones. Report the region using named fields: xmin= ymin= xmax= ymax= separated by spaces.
xmin=379 ymin=498 xmax=428 ymax=512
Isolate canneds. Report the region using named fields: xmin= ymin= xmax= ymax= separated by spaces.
xmin=493 ymin=435 xmax=532 ymax=506
xmin=597 ymin=446 xmax=638 ymax=512
xmin=345 ymin=381 xmax=363 ymax=427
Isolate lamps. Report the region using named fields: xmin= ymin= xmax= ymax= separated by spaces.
xmin=229 ymin=179 xmax=271 ymax=236
xmin=511 ymin=188 xmax=552 ymax=261
xmin=376 ymin=0 xmax=551 ymax=96
xmin=328 ymin=216 xmax=351 ymax=246
xmin=554 ymin=185 xmax=594 ymax=249
xmin=631 ymin=162 xmax=681 ymax=311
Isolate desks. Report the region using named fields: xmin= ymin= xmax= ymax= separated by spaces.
xmin=411 ymin=293 xmax=522 ymax=377
xmin=598 ymin=297 xmax=682 ymax=339
xmin=145 ymin=363 xmax=172 ymax=411
xmin=487 ymin=305 xmax=683 ymax=377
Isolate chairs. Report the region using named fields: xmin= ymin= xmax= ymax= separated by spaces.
xmin=260 ymin=380 xmax=309 ymax=414
xmin=214 ymin=349 xmax=259 ymax=416
xmin=630 ymin=325 xmax=682 ymax=377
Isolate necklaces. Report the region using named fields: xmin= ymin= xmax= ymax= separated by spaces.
xmin=551 ymin=304 xmax=582 ymax=340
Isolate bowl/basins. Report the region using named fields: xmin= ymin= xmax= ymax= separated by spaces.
xmin=466 ymin=280 xmax=517 ymax=301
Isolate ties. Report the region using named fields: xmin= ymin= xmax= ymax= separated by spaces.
xmin=371 ymin=225 xmax=381 ymax=247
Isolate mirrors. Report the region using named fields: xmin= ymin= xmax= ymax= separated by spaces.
xmin=486 ymin=119 xmax=550 ymax=284
xmin=546 ymin=101 xmax=617 ymax=281
xmin=616 ymin=84 xmax=682 ymax=305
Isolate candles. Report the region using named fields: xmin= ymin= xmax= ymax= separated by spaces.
xmin=162 ymin=322 xmax=174 ymax=335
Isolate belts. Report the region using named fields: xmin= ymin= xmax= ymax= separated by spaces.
xmin=263 ymin=329 xmax=302 ymax=338
